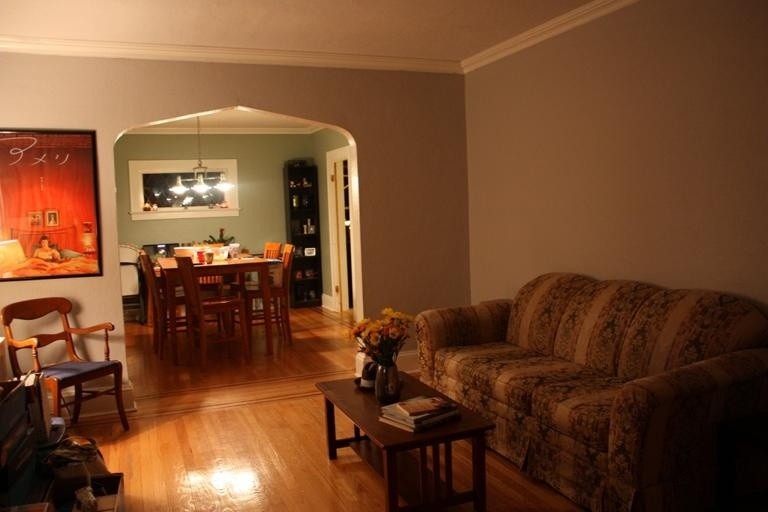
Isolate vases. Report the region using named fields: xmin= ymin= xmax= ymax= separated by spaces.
xmin=373 ymin=358 xmax=401 ymax=407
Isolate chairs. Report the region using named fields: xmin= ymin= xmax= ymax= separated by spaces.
xmin=136 ymin=232 xmax=297 ymax=364
xmin=0 ymin=295 xmax=134 ymax=433
xmin=119 ymin=240 xmax=148 ymax=327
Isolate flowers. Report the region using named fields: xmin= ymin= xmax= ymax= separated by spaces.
xmin=353 ymin=304 xmax=416 ymax=367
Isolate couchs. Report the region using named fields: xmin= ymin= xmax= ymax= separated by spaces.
xmin=410 ymin=271 xmax=768 ymax=512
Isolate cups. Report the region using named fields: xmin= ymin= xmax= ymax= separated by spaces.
xmin=156 ymin=245 xmax=166 ymax=256
xmin=204 ymin=252 xmax=215 ymax=264
xmin=196 ymin=250 xmax=206 ymax=264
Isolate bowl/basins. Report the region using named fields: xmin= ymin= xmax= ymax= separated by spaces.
xmin=173 ymin=246 xmax=194 ymax=257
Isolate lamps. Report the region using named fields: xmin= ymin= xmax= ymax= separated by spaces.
xmin=167 ymin=116 xmax=233 ymax=199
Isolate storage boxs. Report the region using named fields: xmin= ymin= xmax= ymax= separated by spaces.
xmin=42 ymin=467 xmax=127 ymax=512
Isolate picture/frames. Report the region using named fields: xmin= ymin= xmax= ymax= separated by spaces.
xmin=303 ymin=247 xmax=316 ymax=257
xmin=0 ymin=126 xmax=106 ymax=283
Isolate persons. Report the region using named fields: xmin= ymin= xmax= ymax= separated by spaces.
xmin=31 ymin=235 xmax=71 ymax=264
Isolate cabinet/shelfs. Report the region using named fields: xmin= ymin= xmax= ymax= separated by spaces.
xmin=283 ymin=157 xmax=325 ymax=311
xmin=0 ymin=378 xmax=50 ymax=511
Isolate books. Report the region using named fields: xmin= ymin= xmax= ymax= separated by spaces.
xmin=378 ymin=396 xmax=460 ymax=433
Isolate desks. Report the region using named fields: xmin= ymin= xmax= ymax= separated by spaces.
xmin=311 ymin=367 xmax=497 ymax=512
